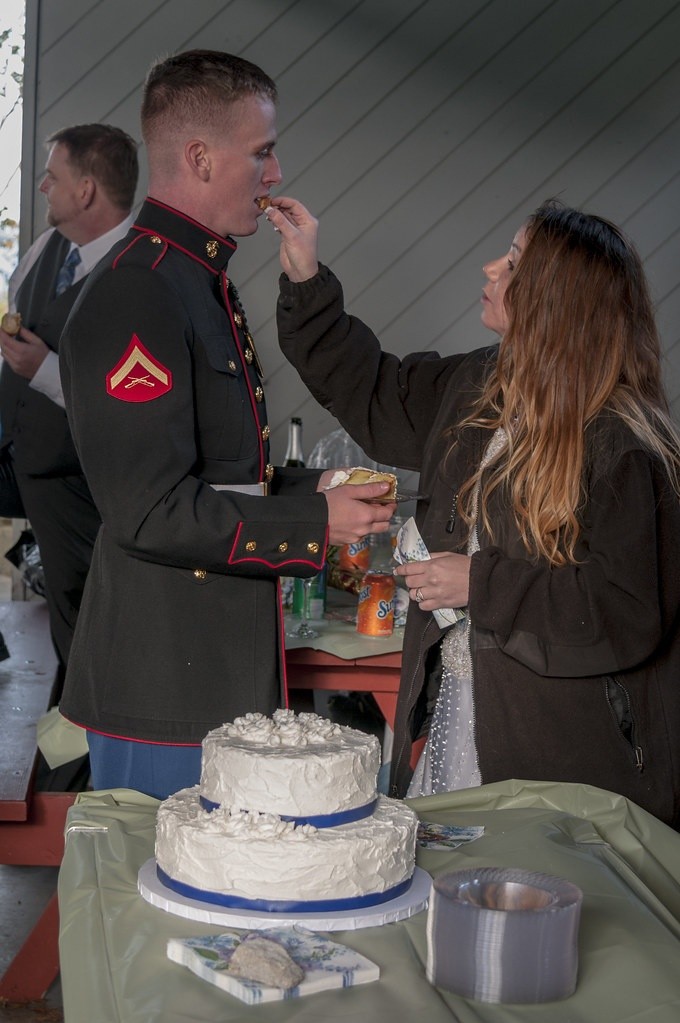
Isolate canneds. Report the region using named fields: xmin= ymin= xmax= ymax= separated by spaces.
xmin=293 ymin=557 xmax=328 ymax=619
xmin=339 ymin=532 xmax=370 ymax=572
xmin=358 ymin=568 xmax=395 ymax=637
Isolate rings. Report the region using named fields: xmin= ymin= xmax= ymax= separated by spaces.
xmin=416 ymin=588 xmax=425 ymax=602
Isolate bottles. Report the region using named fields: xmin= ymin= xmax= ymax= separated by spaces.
xmin=281 ymin=417 xmax=307 ymax=470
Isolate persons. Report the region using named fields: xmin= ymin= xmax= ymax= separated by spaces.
xmin=267 ymin=195 xmax=679 ymax=826
xmin=0 ymin=123 xmax=139 ymax=666
xmin=58 ymin=46 xmax=396 ymax=800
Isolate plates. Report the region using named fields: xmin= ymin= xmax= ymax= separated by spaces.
xmin=302 ymin=484 xmax=432 ymax=505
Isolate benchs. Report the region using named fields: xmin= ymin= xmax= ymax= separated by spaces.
xmin=0 ymin=601 xmax=61 ymax=821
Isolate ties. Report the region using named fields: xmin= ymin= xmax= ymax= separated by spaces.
xmin=55 ymin=248 xmax=81 ymax=296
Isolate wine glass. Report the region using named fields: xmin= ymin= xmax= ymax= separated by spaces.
xmin=285 ymin=575 xmax=322 ymax=640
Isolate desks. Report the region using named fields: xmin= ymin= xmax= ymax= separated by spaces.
xmin=58 ymin=785 xmax=680 ymax=1023
xmin=287 ymin=589 xmax=404 ymax=733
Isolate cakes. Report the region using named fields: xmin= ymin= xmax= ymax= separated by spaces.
xmin=154 ymin=708 xmax=418 ymax=913
xmin=328 ymin=466 xmax=399 ymax=498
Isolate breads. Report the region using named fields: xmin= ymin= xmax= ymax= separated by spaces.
xmin=2 ymin=312 xmax=21 ymax=334
xmin=259 ymin=196 xmax=273 ymax=209
